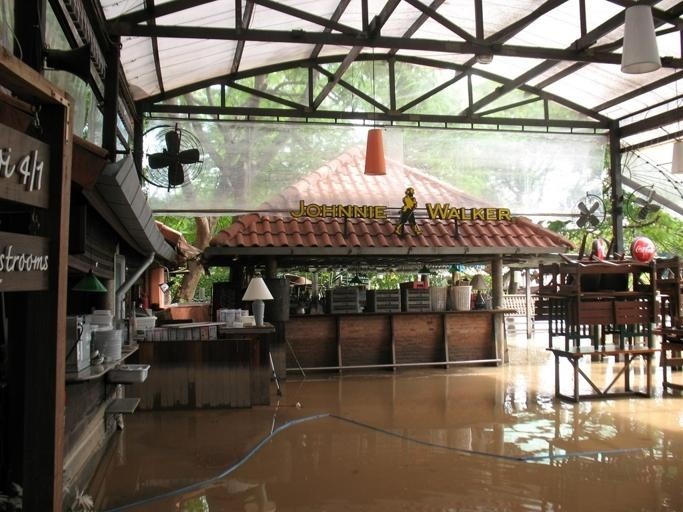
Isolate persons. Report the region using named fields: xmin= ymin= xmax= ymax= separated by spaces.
xmin=394 ymin=188 xmax=424 ymax=235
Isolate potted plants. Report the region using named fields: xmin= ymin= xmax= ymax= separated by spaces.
xmin=291 ymin=291 xmax=312 ymax=315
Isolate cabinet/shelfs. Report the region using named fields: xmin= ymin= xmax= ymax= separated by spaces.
xmin=532 ymin=251 xmax=683 ymax=402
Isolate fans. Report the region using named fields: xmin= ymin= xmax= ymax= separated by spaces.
xmin=620 ymin=184 xmax=665 ymax=225
xmin=105 ymin=122 xmax=207 ymax=192
xmin=571 ymin=195 xmax=623 ymax=232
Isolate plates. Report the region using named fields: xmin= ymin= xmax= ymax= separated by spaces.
xmin=92 ymin=328 xmax=122 ymax=363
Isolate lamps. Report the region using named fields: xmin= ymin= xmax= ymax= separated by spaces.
xmin=241 ymin=278 xmax=275 ymax=328
xmin=363 ymin=36 xmax=387 ymax=176
xmin=70 ymin=247 xmax=108 ymax=293
xmin=418 ymin=262 xmax=430 ymax=274
xmin=470 ymin=274 xmax=489 ymax=309
xmin=349 ymin=263 xmax=362 ymax=284
xmin=670 ymin=67 xmax=683 ymax=175
xmin=620 ymin=0 xmax=662 ymax=74
